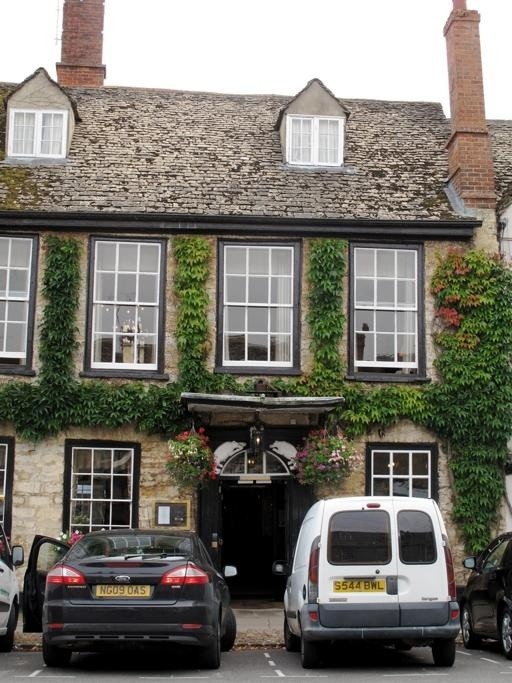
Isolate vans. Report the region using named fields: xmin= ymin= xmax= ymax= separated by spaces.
xmin=271 ymin=494 xmax=463 ymax=668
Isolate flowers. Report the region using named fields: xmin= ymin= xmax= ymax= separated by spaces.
xmin=163 ymin=425 xmax=219 ymax=483
xmin=293 ymin=426 xmax=365 ymax=484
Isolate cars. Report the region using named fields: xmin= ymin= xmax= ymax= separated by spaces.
xmin=460 ymin=530 xmax=512 ymax=659
xmin=21 ymin=528 xmax=238 ymax=670
xmin=0 ymin=523 xmax=24 ymax=654
